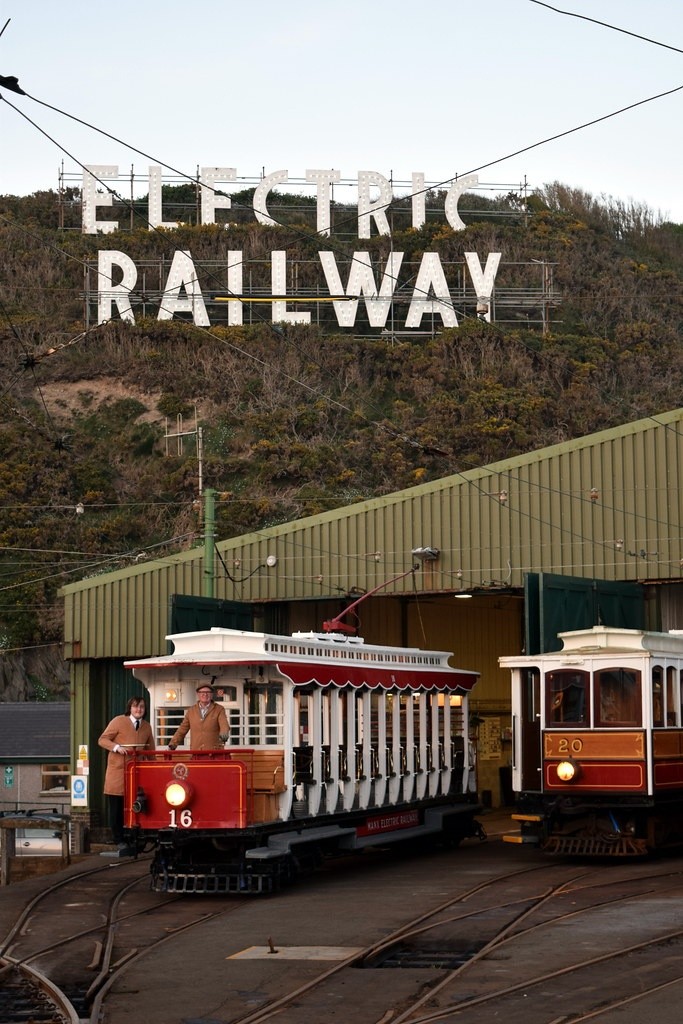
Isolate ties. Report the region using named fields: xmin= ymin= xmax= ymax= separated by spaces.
xmin=135 ymin=720 xmax=140 ymax=732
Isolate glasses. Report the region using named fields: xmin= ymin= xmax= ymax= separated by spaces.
xmin=198 ymin=691 xmax=213 ymax=693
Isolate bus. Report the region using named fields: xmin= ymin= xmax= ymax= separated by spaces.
xmin=112 ymin=622 xmax=481 ymax=896
xmin=493 ymin=624 xmax=683 ymax=865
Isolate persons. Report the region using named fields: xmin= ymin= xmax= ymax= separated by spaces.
xmin=98 ymin=697 xmax=157 ymax=851
xmin=166 ymin=679 xmax=230 ymax=757
